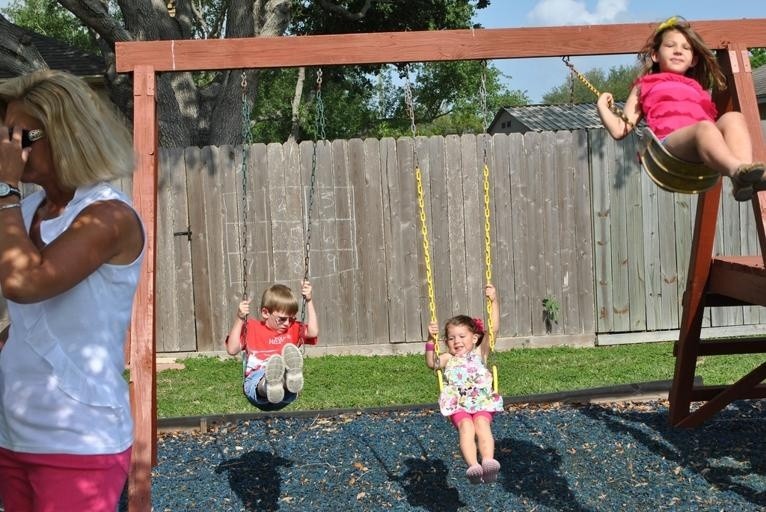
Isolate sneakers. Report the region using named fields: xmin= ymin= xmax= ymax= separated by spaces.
xmin=732 ymin=163 xmax=766 ymax=201
xmin=466 ymin=459 xmax=500 ymax=484
xmin=265 ymin=343 xmax=304 ymax=404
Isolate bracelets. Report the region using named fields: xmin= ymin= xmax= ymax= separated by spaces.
xmin=237 ymin=313 xmax=245 ymax=320
xmin=425 ymin=343 xmax=434 ymax=351
xmin=0 ymin=203 xmax=20 ymax=211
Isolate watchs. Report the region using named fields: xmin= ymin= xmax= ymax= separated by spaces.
xmin=0 ymin=182 xmax=21 ymax=199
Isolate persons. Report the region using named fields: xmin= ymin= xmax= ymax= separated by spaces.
xmin=425 ymin=284 xmax=504 ymax=485
xmin=226 ymin=280 xmax=319 ymax=411
xmin=0 ymin=68 xmax=147 ymax=512
xmin=596 ymin=16 xmax=766 ymax=201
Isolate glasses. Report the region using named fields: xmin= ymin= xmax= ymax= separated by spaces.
xmin=8 ymin=128 xmax=45 ymax=148
xmin=274 ymin=313 xmax=296 ymax=322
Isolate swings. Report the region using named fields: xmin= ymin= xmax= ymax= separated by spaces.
xmin=242 ymin=67 xmax=323 ymax=411
xmin=560 ymin=56 xmax=722 ymax=195
xmin=405 ymin=58 xmax=499 ymax=423
